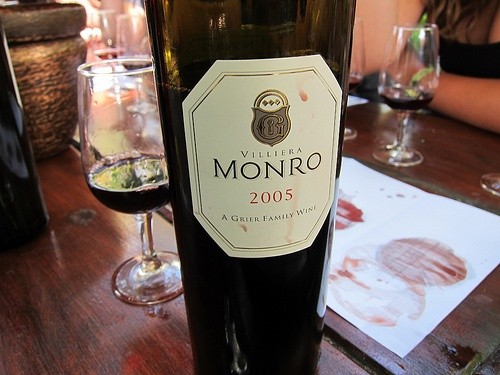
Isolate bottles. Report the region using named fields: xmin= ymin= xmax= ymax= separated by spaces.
xmin=144 ymin=0 xmax=356 ymax=375
xmin=0 ymin=16 xmax=48 ymax=252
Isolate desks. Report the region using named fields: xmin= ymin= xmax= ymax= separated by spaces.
xmin=0 ymin=74 xmax=500 ymax=375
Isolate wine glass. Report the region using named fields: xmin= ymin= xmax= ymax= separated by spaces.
xmin=479 ymin=173 xmax=500 ymax=197
xmin=373 ymin=23 xmax=440 ymax=167
xmin=344 ymin=15 xmax=365 ymax=140
xmin=117 ymin=13 xmax=159 ymax=114
xmin=79 ymin=11 xmax=142 ymax=134
xmin=76 ymin=56 xmax=183 ymax=305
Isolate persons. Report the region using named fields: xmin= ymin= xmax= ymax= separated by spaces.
xmin=392 ymin=0 xmax=500 ymax=134
